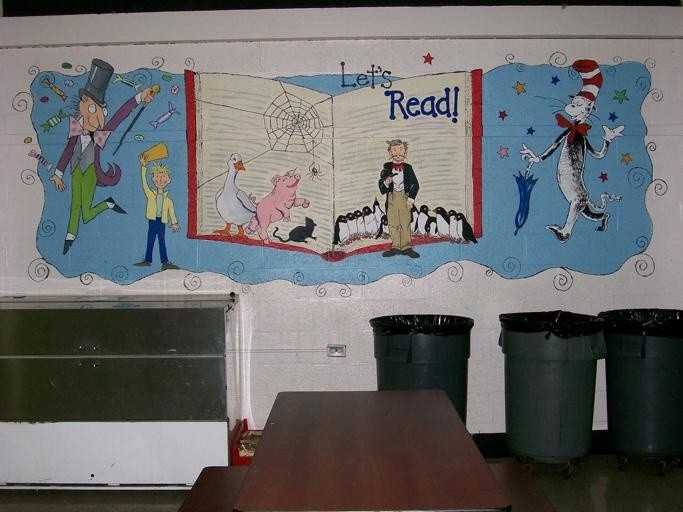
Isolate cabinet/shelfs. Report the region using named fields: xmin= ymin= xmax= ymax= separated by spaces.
xmin=0 ymin=293 xmax=247 ymax=493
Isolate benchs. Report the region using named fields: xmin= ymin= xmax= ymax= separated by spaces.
xmin=484 ymin=455 xmax=556 ymax=512
xmin=174 ymin=465 xmax=249 ymax=512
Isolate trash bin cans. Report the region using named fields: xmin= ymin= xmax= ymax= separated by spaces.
xmin=369 ymin=314 xmax=474 ymax=429
xmin=499 ymin=308 xmax=683 ymax=475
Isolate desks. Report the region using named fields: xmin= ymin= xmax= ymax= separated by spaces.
xmin=233 ymin=388 xmax=511 ymax=512
